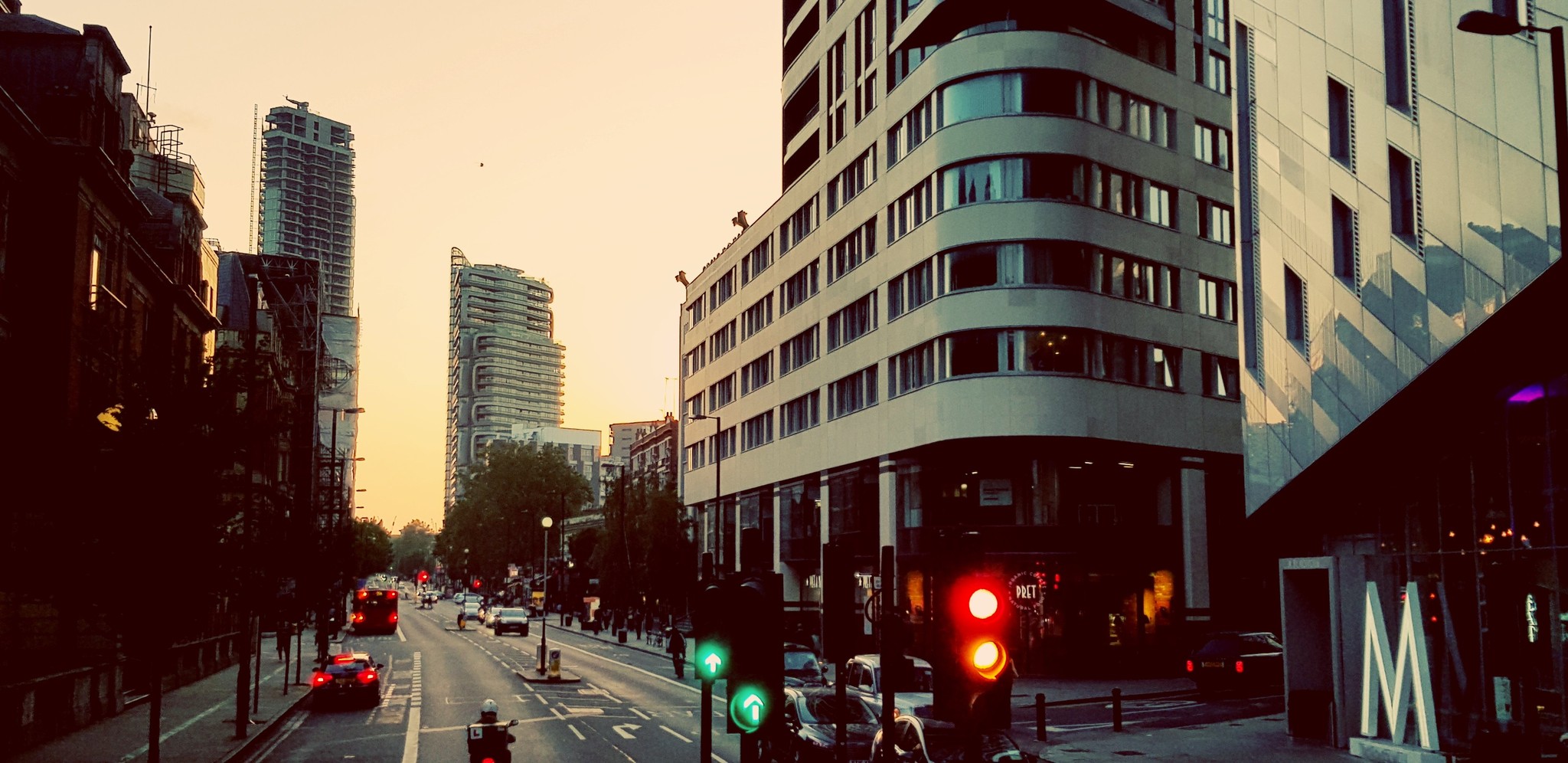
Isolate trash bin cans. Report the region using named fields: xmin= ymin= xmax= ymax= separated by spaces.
xmin=618 ymin=628 xmax=627 ymax=642
xmin=565 ymin=616 xmax=572 ymax=626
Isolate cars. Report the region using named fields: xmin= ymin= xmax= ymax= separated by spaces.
xmin=763 ymin=685 xmax=880 ymax=763
xmin=871 ymin=715 xmax=1030 ymax=762
xmin=311 ymin=652 xmax=385 ymax=707
xmin=485 ymin=606 xmax=500 ymax=628
xmin=494 ymin=607 xmax=531 ymax=636
xmin=452 ymin=592 xmax=492 ymax=605
xmin=458 ymin=601 xmax=482 ymax=622
xmin=778 ymin=641 xmax=830 ymax=690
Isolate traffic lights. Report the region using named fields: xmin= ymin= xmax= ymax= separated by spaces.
xmin=698 ymin=571 xmax=792 ymax=738
xmin=946 ymin=567 xmax=1017 ymax=690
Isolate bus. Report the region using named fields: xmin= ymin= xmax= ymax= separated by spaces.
xmin=351 ymin=587 xmax=399 ymax=634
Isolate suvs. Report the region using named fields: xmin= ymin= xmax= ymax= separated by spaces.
xmin=1184 ymin=632 xmax=1284 ymax=696
xmin=845 ymin=652 xmax=958 ymax=736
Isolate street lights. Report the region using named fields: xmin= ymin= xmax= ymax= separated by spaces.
xmin=1458 ymin=9 xmax=1565 ymax=315
xmin=689 ymin=413 xmax=723 ymax=572
xmin=602 ymin=462 xmax=625 ymax=584
xmin=326 ymin=405 xmax=362 ymax=577
xmin=541 ymin=516 xmax=551 ymax=679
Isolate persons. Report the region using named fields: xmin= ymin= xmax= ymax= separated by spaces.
xmin=427 ymin=594 xmax=434 ymax=609
xmin=594 ymin=605 xmax=654 ymax=645
xmin=315 ymin=623 xmax=330 ymax=665
xmin=466 ymin=698 xmax=516 ymax=762
xmin=668 ymin=625 xmax=687 ymax=676
xmin=276 ymin=612 xmax=290 ymax=663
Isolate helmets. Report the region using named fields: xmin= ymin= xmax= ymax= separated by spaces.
xmin=480 ymin=699 xmax=499 ymax=713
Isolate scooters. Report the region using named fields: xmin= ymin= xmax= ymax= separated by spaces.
xmin=475 ymin=719 xmax=521 ymax=763
